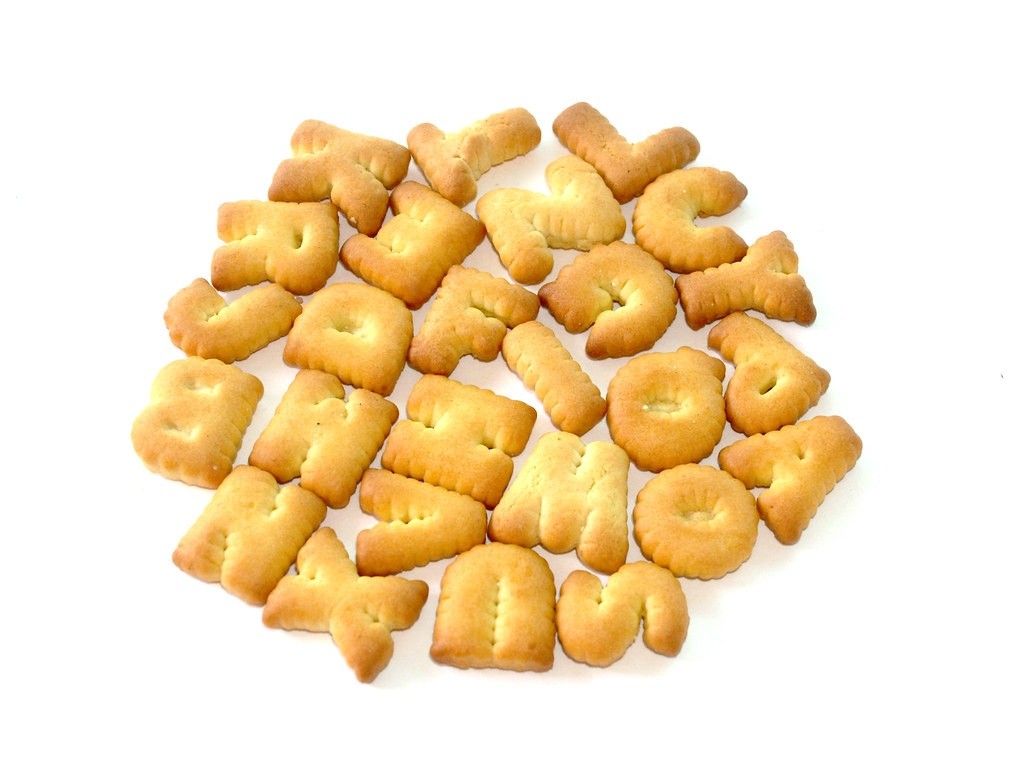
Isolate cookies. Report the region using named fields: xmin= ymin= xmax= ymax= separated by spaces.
xmin=130 ymin=105 xmax=861 ymax=683
